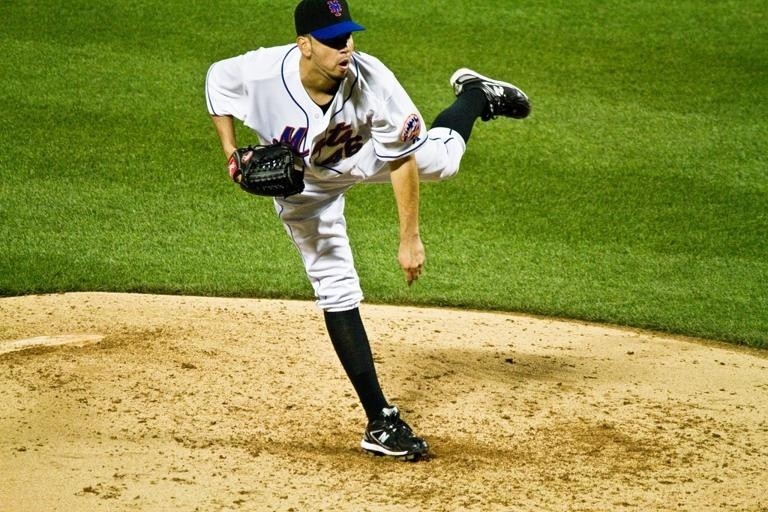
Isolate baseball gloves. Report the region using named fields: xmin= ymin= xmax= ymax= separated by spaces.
xmin=226 ymin=142 xmax=306 ymax=197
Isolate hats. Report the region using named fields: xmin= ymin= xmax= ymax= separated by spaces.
xmin=295 ymin=0 xmax=366 ymax=42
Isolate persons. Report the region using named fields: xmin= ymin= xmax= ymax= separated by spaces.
xmin=205 ymin=2 xmax=531 ymax=460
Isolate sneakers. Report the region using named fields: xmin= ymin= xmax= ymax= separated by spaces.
xmin=361 ymin=404 xmax=429 ymax=460
xmin=449 ymin=68 xmax=532 ymax=121
